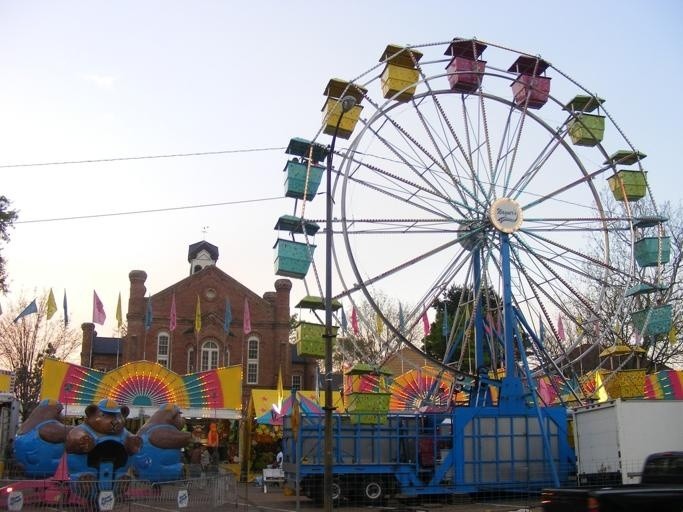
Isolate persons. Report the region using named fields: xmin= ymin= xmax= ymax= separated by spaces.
xmin=208 ymin=423 xmax=218 ymax=447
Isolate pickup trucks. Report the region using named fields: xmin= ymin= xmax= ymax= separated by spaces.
xmin=538 ymin=450 xmax=682 ymax=510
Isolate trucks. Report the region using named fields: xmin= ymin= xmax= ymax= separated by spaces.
xmin=572 ymin=400 xmax=681 ymax=490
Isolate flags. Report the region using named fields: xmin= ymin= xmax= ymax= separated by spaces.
xmin=192 ymin=293 xmax=205 ymax=335
xmin=352 ymin=309 xmax=359 ymax=336
xmin=596 ymin=319 xmax=656 ymax=347
xmin=539 ymin=313 xmax=583 ymax=343
xmin=399 ymin=307 xmax=404 ymax=334
xmin=145 ymin=295 xmax=154 ymax=331
xmin=167 ymin=291 xmax=178 ymax=331
xmin=91 ymin=290 xmax=107 ymax=326
xmin=241 ymin=296 xmax=254 ymax=334
xmin=45 ymin=287 xmax=58 ymax=321
xmin=222 ymin=294 xmax=233 ymax=333
xmin=115 ymin=291 xmax=123 ymax=330
xmin=443 ymin=308 xmax=449 ymax=336
xmin=377 ymin=314 xmax=384 ymax=334
xmin=11 ymin=298 xmax=39 ymax=325
xmin=423 ymin=313 xmax=430 ymax=336
xmin=342 ymin=311 xmax=348 ymax=333
xmin=62 ymin=291 xmax=69 ymax=328
xmin=667 ymin=324 xmax=677 ymax=346
xmin=466 ymin=307 xmax=501 ymax=341
